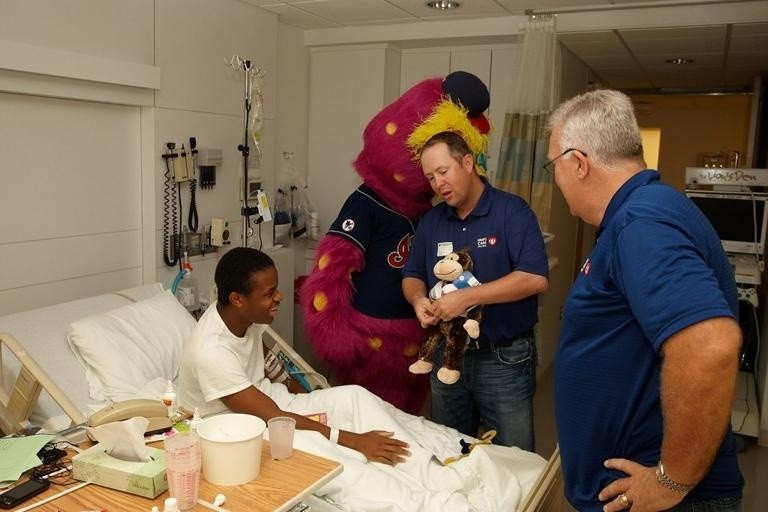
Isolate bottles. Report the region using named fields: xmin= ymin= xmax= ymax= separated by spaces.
xmin=163 ymin=380 xmax=178 ymax=417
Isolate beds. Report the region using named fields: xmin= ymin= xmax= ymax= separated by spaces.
xmin=0 ymin=280 xmax=563 ymax=512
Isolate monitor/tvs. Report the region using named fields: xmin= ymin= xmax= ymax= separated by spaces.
xmin=686 ymin=192 xmax=768 ymax=255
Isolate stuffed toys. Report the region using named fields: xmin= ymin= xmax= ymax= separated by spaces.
xmin=407 ymin=248 xmax=485 ymax=385
xmin=297 ymin=70 xmax=493 ymax=418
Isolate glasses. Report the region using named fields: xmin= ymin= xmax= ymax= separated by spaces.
xmin=544 ymin=149 xmax=589 ymax=174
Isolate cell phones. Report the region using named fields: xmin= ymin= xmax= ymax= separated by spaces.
xmin=0 ymin=475 xmax=51 ymax=509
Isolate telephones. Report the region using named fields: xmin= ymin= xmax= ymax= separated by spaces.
xmin=86 ymin=399 xmax=173 ymax=443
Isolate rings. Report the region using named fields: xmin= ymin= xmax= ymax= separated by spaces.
xmin=618 ymin=493 xmax=629 ymax=505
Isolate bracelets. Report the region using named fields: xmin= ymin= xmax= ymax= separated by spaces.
xmin=328 ymin=425 xmax=341 ymax=446
xmin=654 ymin=458 xmax=698 ymax=492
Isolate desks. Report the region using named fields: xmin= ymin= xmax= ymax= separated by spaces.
xmin=2 ymin=419 xmax=345 ymax=512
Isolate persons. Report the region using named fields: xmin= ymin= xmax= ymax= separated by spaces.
xmin=540 ymin=88 xmax=747 ymax=512
xmin=401 ymin=131 xmax=550 ymax=453
xmin=175 ymin=246 xmax=411 ymax=468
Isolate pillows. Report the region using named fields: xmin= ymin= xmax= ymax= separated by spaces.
xmin=69 ymin=288 xmax=197 ymax=396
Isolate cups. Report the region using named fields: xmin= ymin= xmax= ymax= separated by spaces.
xmin=163 ymin=431 xmax=202 ymax=511
xmin=267 ymin=415 xmax=296 ymax=461
xmin=195 ymin=412 xmax=267 ymax=487
